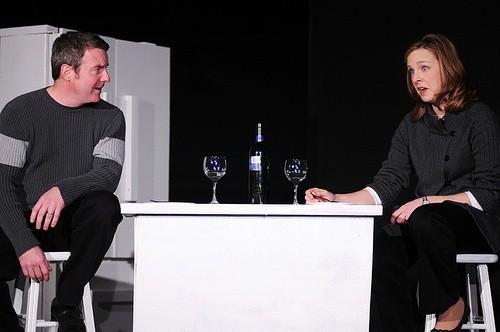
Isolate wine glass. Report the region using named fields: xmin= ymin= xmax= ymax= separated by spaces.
xmin=284 ymin=159 xmax=308 ymax=205
xmin=203 ymin=155 xmax=227 ymax=204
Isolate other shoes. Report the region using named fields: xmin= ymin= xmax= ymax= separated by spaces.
xmin=431 ymin=299 xmax=470 ymax=332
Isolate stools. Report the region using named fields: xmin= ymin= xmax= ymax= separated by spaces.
xmin=13 ymin=251 xmax=97 ymax=332
xmin=424 ymin=253 xmax=500 ymax=332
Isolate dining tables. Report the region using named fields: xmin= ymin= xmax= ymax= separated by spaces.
xmin=119 ymin=201 xmax=384 ymax=332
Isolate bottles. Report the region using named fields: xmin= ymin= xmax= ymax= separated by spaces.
xmin=249 ymin=123 xmax=271 ymax=204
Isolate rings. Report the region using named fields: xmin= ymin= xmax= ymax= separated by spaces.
xmin=48 ymin=212 xmax=53 ymax=215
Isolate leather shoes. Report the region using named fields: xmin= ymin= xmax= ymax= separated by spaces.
xmin=50 ymin=297 xmax=86 ymax=332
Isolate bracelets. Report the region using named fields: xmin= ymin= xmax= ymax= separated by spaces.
xmin=421 ymin=196 xmax=430 ymax=205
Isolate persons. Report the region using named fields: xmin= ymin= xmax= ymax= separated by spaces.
xmin=304 ymin=34 xmax=500 ymax=332
xmin=0 ymin=31 xmax=126 ymax=332
